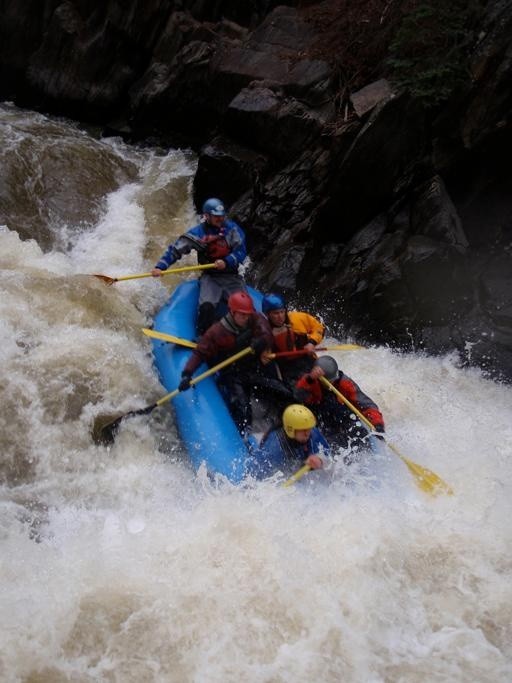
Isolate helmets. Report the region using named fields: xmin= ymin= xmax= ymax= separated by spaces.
xmin=261 ymin=294 xmax=286 ymax=313
xmin=202 ymin=197 xmax=226 ymax=216
xmin=313 ymin=355 xmax=340 ymax=384
xmin=281 ymin=403 xmax=317 ymax=439
xmin=227 ymin=291 xmax=257 ymax=314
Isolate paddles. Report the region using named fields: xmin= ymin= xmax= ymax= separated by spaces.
xmin=141 ymin=327 xmax=199 ymax=352
xmin=266 ymin=343 xmax=361 ymax=359
xmin=319 ymin=376 xmax=454 ymax=496
xmin=73 ymin=263 xmax=218 ymax=287
xmin=102 ymin=346 xmax=256 ymax=442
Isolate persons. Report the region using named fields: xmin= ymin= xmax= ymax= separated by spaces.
xmin=293 ymin=355 xmax=385 ymax=454
xmin=262 ymin=293 xmax=326 ymax=384
xmin=178 ymin=292 xmax=294 ymax=448
xmin=151 ymin=198 xmax=247 ymax=341
xmin=257 ymin=403 xmax=332 ymax=484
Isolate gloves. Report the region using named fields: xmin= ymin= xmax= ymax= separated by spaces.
xmin=178 ymin=376 xmax=196 ymax=393
xmin=251 ymin=338 xmax=267 ymax=354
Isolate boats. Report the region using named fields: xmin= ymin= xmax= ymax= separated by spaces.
xmin=142 ymin=275 xmax=389 ymax=496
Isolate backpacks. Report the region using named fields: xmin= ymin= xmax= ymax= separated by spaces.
xmin=182 ymin=226 xmax=230 ymax=275
